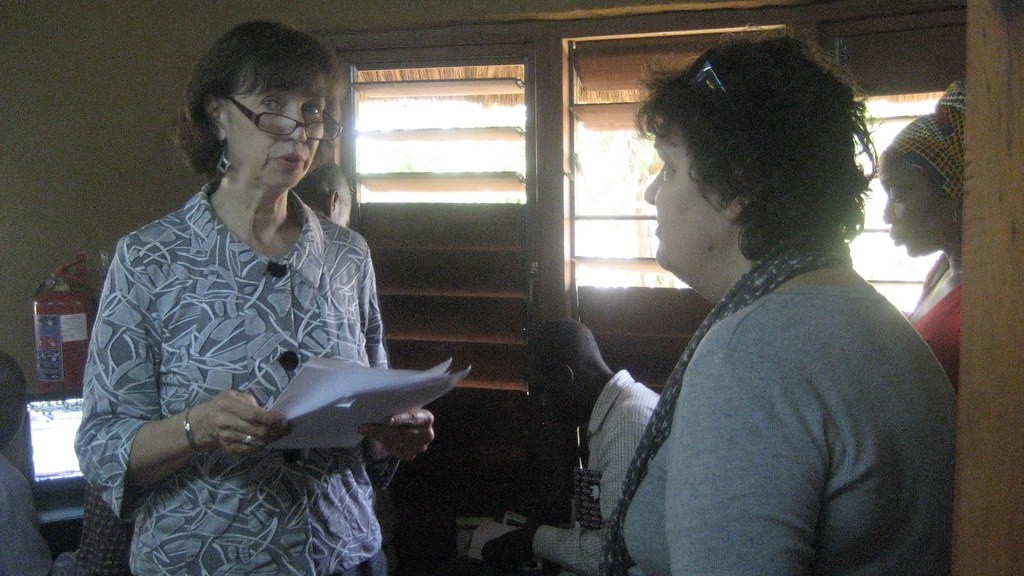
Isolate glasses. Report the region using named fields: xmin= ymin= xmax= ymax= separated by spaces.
xmin=221 ymin=91 xmax=343 ymax=142
xmin=683 ymin=47 xmax=738 ymax=115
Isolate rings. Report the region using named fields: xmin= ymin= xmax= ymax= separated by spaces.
xmin=245 ymin=434 xmax=254 ymax=445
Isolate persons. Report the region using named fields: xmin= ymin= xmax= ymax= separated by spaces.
xmin=75 ymin=20 xmax=436 ymax=576
xmin=0 ymin=33 xmax=966 ymax=575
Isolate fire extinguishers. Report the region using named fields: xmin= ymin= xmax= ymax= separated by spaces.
xmin=31 ymin=252 xmax=96 ymax=401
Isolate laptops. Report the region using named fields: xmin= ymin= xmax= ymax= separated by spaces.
xmin=28 ymin=391 xmax=90 ymax=527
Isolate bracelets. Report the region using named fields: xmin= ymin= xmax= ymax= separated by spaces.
xmin=183 ymin=406 xmax=206 ymax=455
